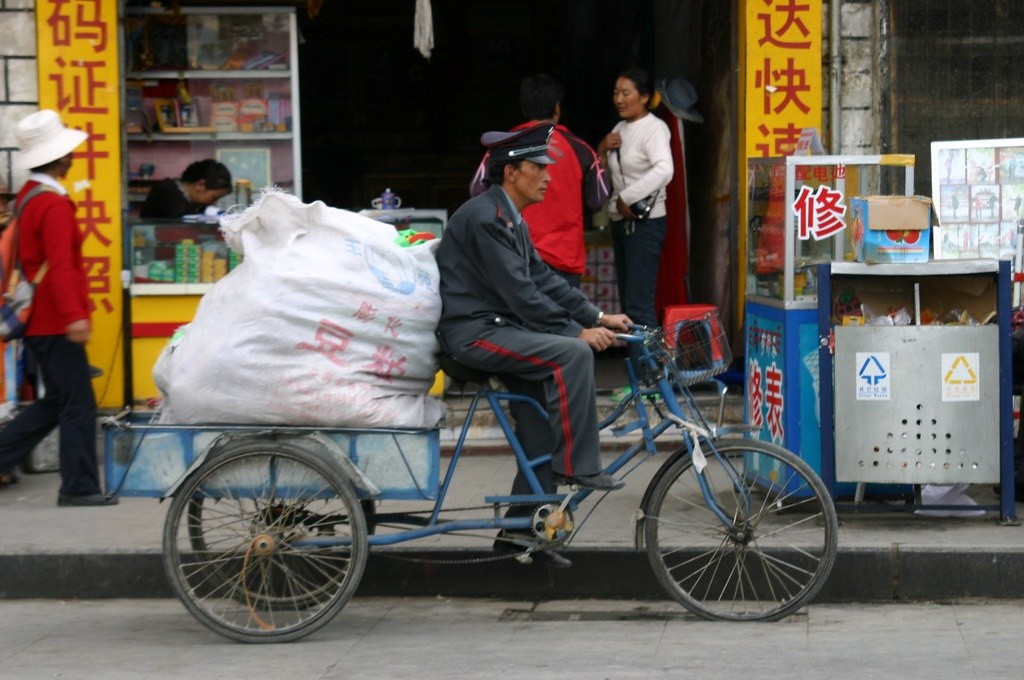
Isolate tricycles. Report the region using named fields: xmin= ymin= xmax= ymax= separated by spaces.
xmin=99 ymin=323 xmax=838 ymax=644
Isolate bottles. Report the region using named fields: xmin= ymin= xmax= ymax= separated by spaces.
xmin=132 ymin=230 xmax=146 ymax=266
xmin=236 ymin=180 xmax=251 ymax=210
xmin=382 ymin=188 xmax=396 ymax=210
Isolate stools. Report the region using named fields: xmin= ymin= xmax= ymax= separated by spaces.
xmin=658 ymin=304 xmax=726 ymax=396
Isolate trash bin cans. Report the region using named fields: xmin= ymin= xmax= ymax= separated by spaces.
xmin=816 ymin=256 xmax=1016 ymax=521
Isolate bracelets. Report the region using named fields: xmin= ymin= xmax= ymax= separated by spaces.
xmin=597 ymin=309 xmax=605 ymax=325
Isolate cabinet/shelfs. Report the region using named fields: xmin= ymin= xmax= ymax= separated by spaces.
xmin=122 ymin=9 xmax=300 ymax=225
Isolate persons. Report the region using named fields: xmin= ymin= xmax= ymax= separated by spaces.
xmin=468 ymin=74 xmax=611 ymax=288
xmin=139 ymin=159 xmax=234 ymax=225
xmin=436 ymin=122 xmax=635 ymax=571
xmin=0 ymin=108 xmax=118 ymax=507
xmin=597 ymin=72 xmax=675 ymax=400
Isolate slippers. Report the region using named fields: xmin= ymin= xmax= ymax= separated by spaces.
xmin=610 ymin=383 xmax=662 ymax=401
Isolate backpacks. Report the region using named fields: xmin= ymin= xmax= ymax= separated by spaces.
xmin=0 ymin=186 xmax=63 ymax=342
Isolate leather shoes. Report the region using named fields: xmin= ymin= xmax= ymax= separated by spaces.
xmin=57 ymin=487 xmax=119 ymax=508
xmin=549 ymin=467 xmax=625 ymax=490
xmin=493 ymin=532 xmax=571 ymax=567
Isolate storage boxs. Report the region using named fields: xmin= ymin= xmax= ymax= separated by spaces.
xmin=128 ymin=29 xmax=288 ymax=284
xmin=854 ymin=193 xmax=932 ymax=263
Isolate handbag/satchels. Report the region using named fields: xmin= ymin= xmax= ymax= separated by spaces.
xmin=630 ymin=191 xmax=658 ymax=220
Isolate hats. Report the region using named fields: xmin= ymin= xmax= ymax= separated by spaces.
xmin=14 ymin=109 xmax=91 ymax=169
xmin=481 ymin=122 xmax=557 ymax=168
